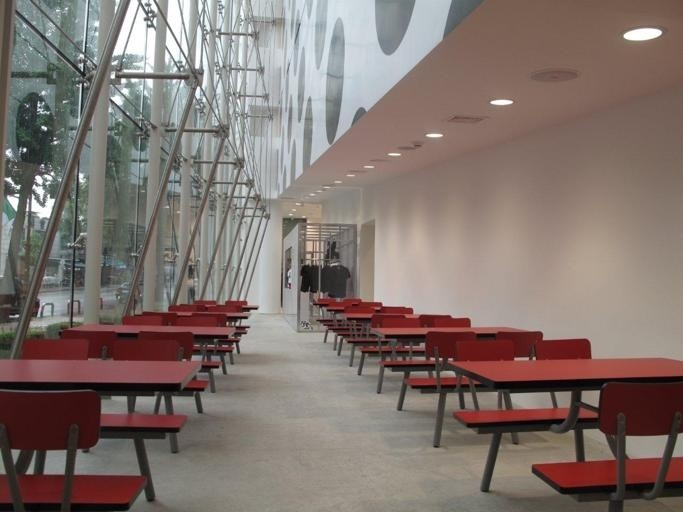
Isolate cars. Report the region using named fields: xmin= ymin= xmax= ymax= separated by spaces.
xmin=113 ymin=282 xmax=139 ymax=303
